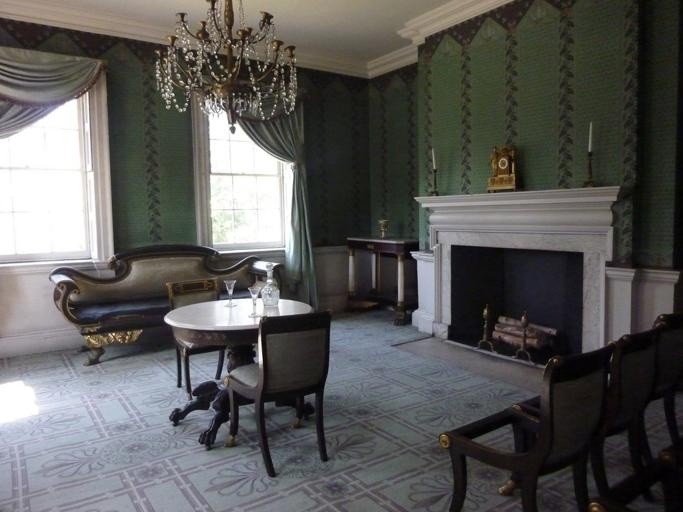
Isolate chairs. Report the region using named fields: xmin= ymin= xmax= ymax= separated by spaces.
xmin=223 ymin=308 xmax=333 ymax=478
xmin=157 ymin=275 xmax=227 ymax=402
xmin=437 ymin=309 xmax=682 ymax=511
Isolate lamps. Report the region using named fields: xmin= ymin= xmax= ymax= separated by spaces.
xmin=153 ymin=0 xmax=300 ymax=135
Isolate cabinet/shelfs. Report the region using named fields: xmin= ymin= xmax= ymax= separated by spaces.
xmin=346 ymin=238 xmax=418 ymax=325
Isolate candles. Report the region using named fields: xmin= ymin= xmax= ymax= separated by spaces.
xmin=431 ymin=148 xmax=436 ymax=171
xmin=587 ymin=122 xmax=592 ymax=153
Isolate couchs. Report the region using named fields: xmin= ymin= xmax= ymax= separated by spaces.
xmin=47 ymin=243 xmax=285 ymax=366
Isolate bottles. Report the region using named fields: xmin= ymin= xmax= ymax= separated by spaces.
xmin=261 ymin=264 xmax=280 ymax=309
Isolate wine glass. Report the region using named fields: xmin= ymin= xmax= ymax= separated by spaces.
xmin=247 ymin=287 xmax=262 ymax=318
xmin=223 ymin=280 xmax=237 ymax=307
